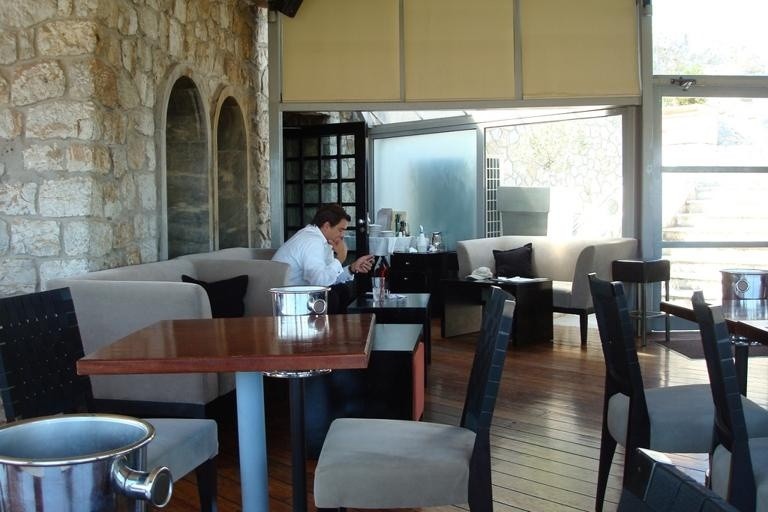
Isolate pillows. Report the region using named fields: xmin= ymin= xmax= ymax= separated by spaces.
xmin=179 ymin=272 xmax=251 ymax=317
xmin=491 ymin=240 xmax=535 ymax=276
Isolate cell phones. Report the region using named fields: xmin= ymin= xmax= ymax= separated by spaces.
xmin=360 ymin=256 xmax=374 ymax=267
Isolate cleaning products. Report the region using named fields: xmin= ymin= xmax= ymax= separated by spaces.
xmin=417 ymin=224 xmax=427 ymax=252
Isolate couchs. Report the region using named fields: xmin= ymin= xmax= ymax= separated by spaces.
xmin=453 ymin=229 xmax=639 ymax=353
xmin=69 ymin=246 xmax=290 ymax=406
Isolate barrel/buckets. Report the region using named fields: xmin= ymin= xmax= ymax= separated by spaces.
xmin=720 ymin=269 xmax=768 ymax=299
xmin=266 ymin=284 xmax=331 ymax=315
xmin=0 ymin=412 xmax=174 ymax=511
xmin=272 ymin=314 xmax=330 ymax=344
xmin=722 ymin=300 xmax=768 ymax=320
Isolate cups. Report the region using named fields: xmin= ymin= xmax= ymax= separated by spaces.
xmin=371 ymin=277 xmax=386 ymax=302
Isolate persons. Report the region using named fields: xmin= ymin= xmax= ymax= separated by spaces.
xmin=271 ymin=203 xmax=376 ymax=314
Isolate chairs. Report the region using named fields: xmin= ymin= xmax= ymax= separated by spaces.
xmin=1 ymin=285 xmax=223 ymax=511
xmin=307 ymin=285 xmax=517 ymax=510
xmin=581 ymin=269 xmax=768 ymax=511
xmin=691 ymin=288 xmax=768 ymax=512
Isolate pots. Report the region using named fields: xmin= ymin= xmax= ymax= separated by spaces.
xmin=720 ymin=269 xmax=768 ymax=345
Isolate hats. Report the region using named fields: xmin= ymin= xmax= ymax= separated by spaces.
xmin=465 ymin=267 xmax=493 ymax=280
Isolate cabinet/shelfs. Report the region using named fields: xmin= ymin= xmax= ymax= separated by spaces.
xmin=391 ymin=252 xmax=458 ymax=287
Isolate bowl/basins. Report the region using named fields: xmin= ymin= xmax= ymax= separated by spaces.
xmin=344 ymin=224 xmax=440 ymax=256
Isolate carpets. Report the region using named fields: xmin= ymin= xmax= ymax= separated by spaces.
xmin=650 ymin=336 xmax=768 ymax=359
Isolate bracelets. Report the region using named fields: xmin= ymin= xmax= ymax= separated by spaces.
xmin=348 ymin=266 xmax=355 ymax=274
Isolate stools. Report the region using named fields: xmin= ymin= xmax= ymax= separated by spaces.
xmin=608 ymin=258 xmax=672 ymax=350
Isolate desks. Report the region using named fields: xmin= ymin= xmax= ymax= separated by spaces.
xmin=338 ymin=291 xmax=435 ymax=364
xmin=437 ymin=274 xmax=555 ymax=348
xmin=342 ymin=234 xmax=416 ymax=267
xmin=337 ymin=322 xmax=426 ymax=419
